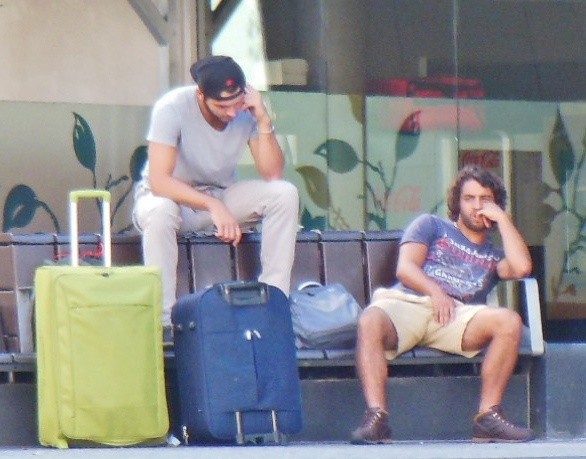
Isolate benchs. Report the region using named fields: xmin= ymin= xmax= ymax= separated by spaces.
xmin=0 ymin=228 xmax=548 ymax=441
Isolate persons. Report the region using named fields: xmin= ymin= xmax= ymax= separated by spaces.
xmin=132 ymin=55 xmax=301 ymax=351
xmin=350 ymin=165 xmax=535 ymax=445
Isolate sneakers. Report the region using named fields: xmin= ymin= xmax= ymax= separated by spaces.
xmin=163 ymin=326 xmax=174 ymax=348
xmin=472 ymin=404 xmax=536 ymax=443
xmin=350 ymin=406 xmax=393 ymax=445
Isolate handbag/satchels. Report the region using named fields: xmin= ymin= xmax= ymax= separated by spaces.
xmin=289 ymin=280 xmax=362 ymax=350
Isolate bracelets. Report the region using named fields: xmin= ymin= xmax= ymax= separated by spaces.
xmin=256 ymin=126 xmax=275 ymax=134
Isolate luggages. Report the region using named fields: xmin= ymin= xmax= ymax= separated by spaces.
xmin=171 ymin=279 xmax=306 ymax=446
xmin=34 ymin=190 xmax=170 ymax=449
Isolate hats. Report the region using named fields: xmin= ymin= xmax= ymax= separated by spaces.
xmin=190 ymin=55 xmax=246 ymax=101
